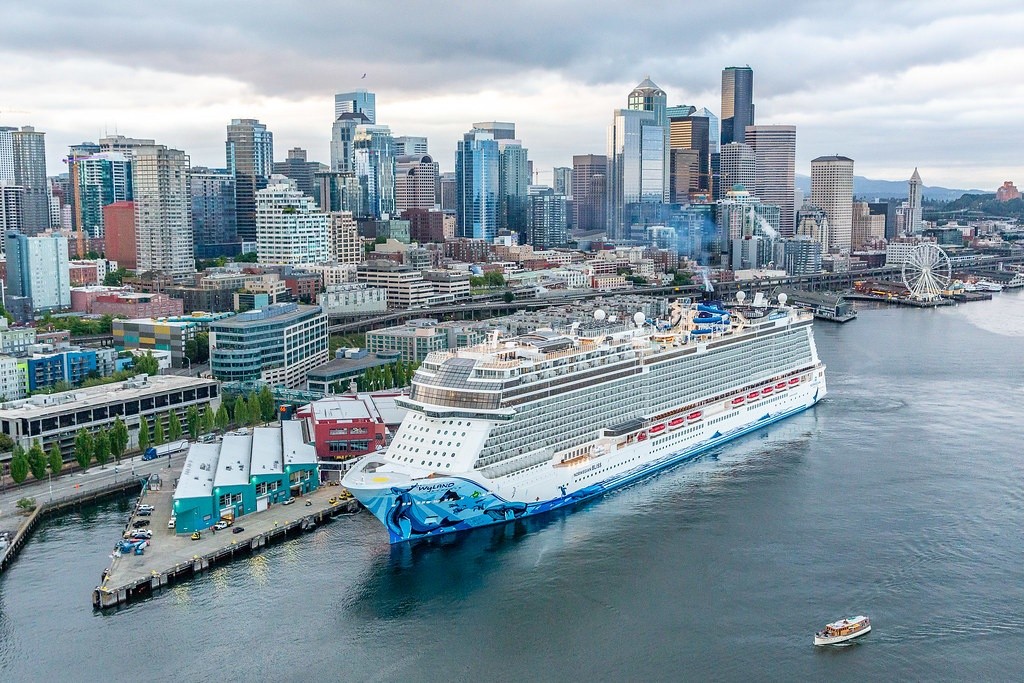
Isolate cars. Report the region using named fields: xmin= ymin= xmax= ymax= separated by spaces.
xmin=283 ymin=496 xmax=295 ymax=505
xmin=118 ymin=503 xmax=155 ymax=556
xmin=232 ymin=527 xmax=245 ymax=534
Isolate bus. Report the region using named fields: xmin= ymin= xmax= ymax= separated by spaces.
xmin=197 ymin=432 xmax=217 ymax=445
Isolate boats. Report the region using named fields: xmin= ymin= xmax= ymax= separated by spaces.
xmin=812 ymin=615 xmax=872 ymax=646
xmin=686 ymin=410 xmax=703 ymax=424
xmin=648 ymin=424 xmax=666 ymax=437
xmin=788 ymin=378 xmax=800 ymax=388
xmin=760 ymin=386 xmax=775 ymax=398
xmin=746 ymin=391 xmax=761 ymax=403
xmin=948 ymin=264 xmax=1024 ymax=293
xmin=666 ymin=417 xmax=685 ymax=431
xmin=774 ymin=382 xmax=787 ymax=393
xmin=732 ymin=396 xmax=745 ymax=409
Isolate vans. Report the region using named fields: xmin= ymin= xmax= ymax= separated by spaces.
xmin=214 ymin=520 xmax=228 ymax=530
xmin=168 ymin=519 xmax=177 ymax=529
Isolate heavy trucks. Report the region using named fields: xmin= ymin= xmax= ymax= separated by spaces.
xmin=142 ymin=439 xmax=189 ymax=461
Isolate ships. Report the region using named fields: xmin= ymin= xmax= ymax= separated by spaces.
xmin=339 ymin=290 xmax=829 ymax=547
